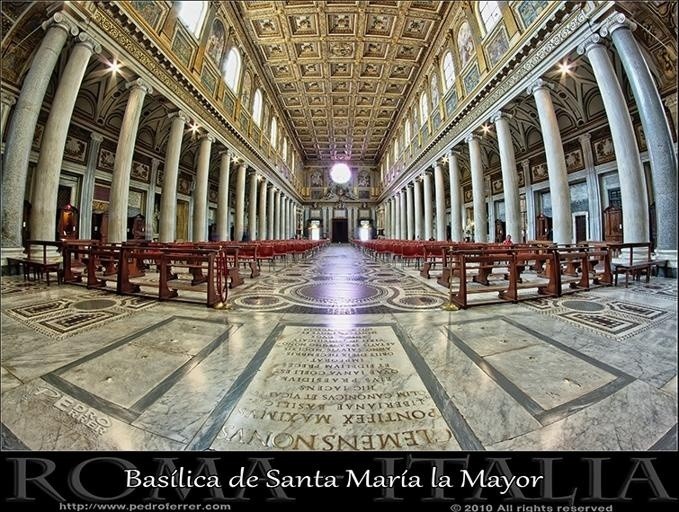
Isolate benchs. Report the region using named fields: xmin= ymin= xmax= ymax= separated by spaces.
xmin=420 ymin=243 xmax=669 ymax=309
xmin=7 ymin=240 xmax=261 ymax=309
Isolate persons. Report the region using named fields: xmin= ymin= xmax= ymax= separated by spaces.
xmin=502 ymin=235 xmax=514 ymax=265
xmin=428 ymin=236 xmax=435 ymax=241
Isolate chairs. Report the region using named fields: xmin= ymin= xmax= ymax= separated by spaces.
xmin=350 ymin=239 xmax=512 ymax=271
xmin=147 ymin=239 xmax=329 ymax=272
